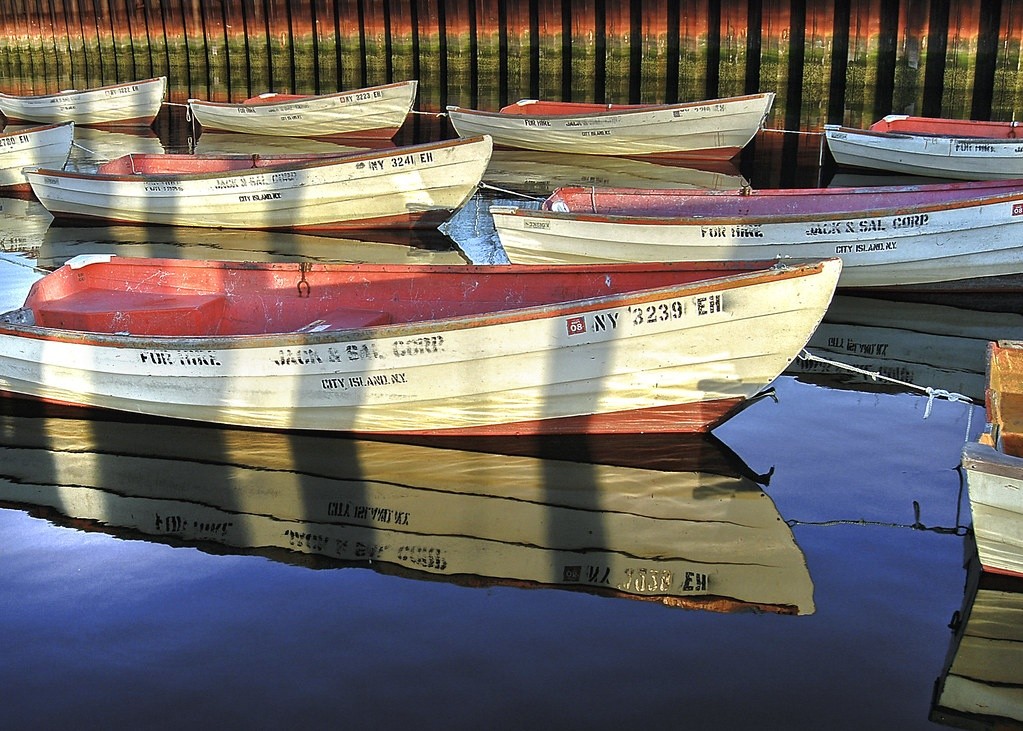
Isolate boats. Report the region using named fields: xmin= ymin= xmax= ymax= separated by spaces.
xmin=822 ymin=113 xmax=1023 ymax=182
xmin=0 ymin=119 xmax=76 ymax=189
xmin=480 ymin=177 xmax=1023 ymax=290
xmin=187 ymin=79 xmax=419 ymax=140
xmin=958 ymin=338 xmax=1023 ymax=580
xmin=0 ymin=252 xmax=843 ymax=437
xmin=0 ymin=75 xmax=168 ymax=127
xmin=20 ymin=132 xmax=494 ymax=232
xmin=1 ymin=412 xmax=820 ymax=624
xmin=446 ymin=92 xmax=779 ymax=162
xmin=916 ymin=518 xmax=1023 ymax=730
xmin=779 ymin=287 xmax=1023 ymax=410
